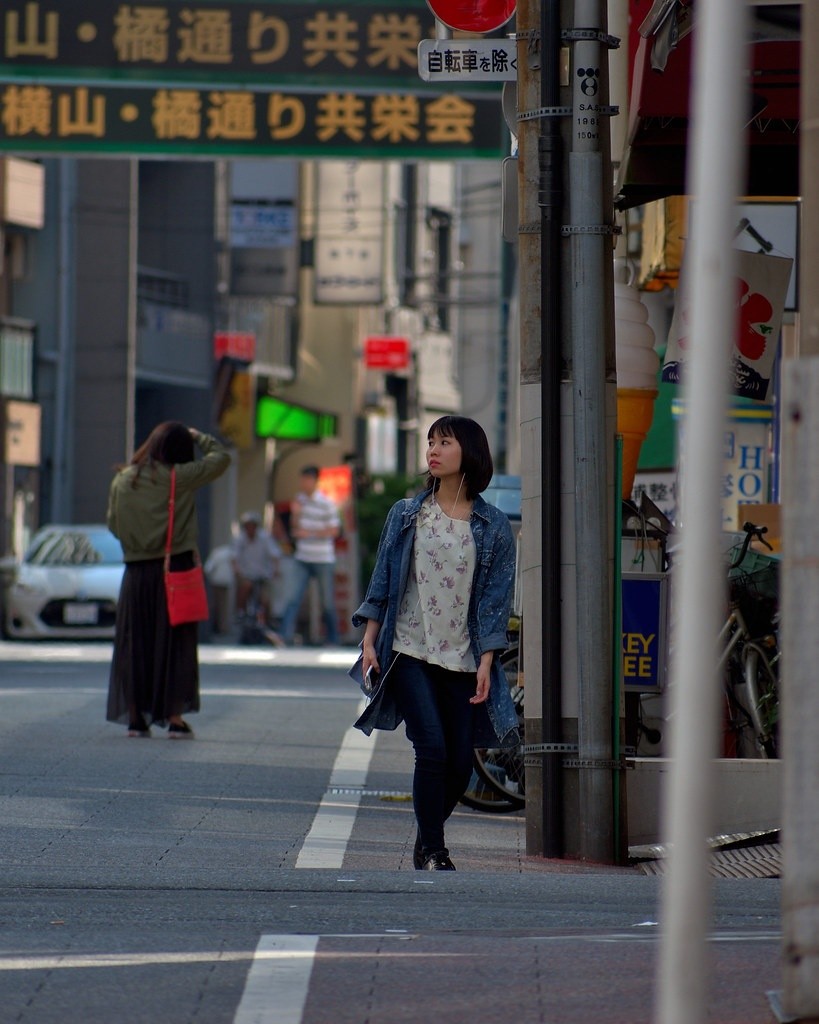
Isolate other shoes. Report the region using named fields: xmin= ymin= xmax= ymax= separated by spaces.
xmin=168 ymin=721 xmax=194 ymax=739
xmin=128 ymin=718 xmax=151 ymax=737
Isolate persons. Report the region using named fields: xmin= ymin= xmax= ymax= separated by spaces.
xmin=348 ymin=415 xmax=517 ymax=872
xmin=271 ymin=463 xmax=341 ymax=648
xmin=229 ymin=511 xmax=280 ymax=626
xmin=107 ymin=419 xmax=231 ymax=738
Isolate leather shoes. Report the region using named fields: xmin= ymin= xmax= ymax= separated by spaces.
xmin=421 ymin=851 xmax=456 ymax=871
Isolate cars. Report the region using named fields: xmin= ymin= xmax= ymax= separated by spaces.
xmin=7 ymin=523 xmax=125 ymax=640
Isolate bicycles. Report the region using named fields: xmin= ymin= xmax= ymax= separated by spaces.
xmin=444 ymin=520 xmax=781 ymax=811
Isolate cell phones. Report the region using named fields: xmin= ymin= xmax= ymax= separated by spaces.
xmin=366 ymin=665 xmax=377 ymax=689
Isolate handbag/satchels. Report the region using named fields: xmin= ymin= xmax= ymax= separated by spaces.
xmin=164 ymin=566 xmax=209 ymax=626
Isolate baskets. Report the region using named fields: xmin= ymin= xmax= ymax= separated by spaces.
xmin=728 ymin=561 xmax=779 ymax=613
